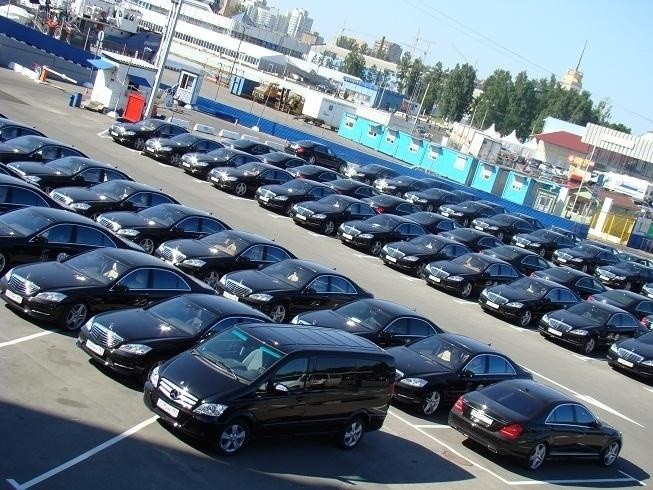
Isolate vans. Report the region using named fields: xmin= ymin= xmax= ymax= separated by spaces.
xmin=135 ymin=318 xmax=398 ymax=459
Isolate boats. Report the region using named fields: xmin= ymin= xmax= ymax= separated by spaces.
xmin=0 ymin=0 xmax=163 ymax=65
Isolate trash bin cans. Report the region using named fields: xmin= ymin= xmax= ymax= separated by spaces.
xmin=69 ymin=93 xmax=82 ymax=108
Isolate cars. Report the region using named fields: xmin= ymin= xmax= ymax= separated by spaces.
xmin=440 ymin=375 xmax=627 ymax=474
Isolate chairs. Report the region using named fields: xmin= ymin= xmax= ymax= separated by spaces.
xmin=438 ymin=350 xmax=454 ymax=364
xmin=526 ymin=284 xmax=536 ymax=293
xmin=426 ymin=241 xmax=435 ymax=251
xmin=186 ymin=309 xmax=209 ymax=331
xmin=288 ymin=269 xmax=303 ymax=282
xmin=582 ymin=311 xmax=592 ymax=320
xmin=226 ymin=240 xmax=240 ymax=252
xmin=242 ymin=347 xmax=281 ymax=373
xmin=103 ymin=261 xmax=121 ymax=280
xmin=333 ymin=201 xmax=339 ymax=207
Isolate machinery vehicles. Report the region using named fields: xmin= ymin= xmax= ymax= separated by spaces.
xmin=274 ymin=86 xmax=306 ymax=116
xmin=249 ymin=79 xmax=281 ymax=106
